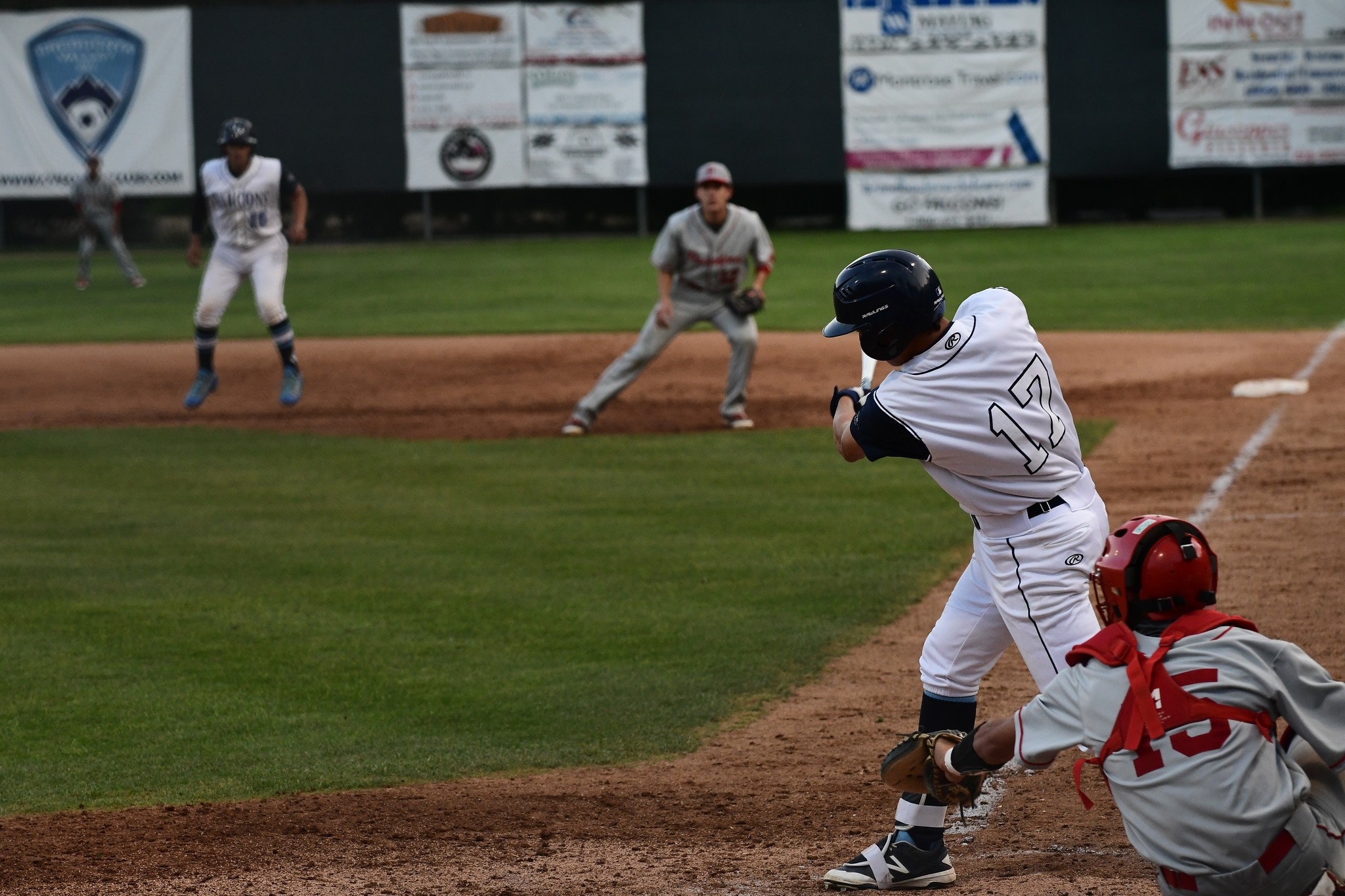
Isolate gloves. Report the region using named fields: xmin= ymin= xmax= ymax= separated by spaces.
xmin=854 ymin=392 xmax=871 ymax=412
xmin=830 ymin=385 xmax=865 ymax=418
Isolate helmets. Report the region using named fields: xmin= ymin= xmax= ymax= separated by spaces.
xmin=219 ymin=118 xmax=257 ymax=152
xmin=1090 ymin=514 xmax=1217 ymax=623
xmin=822 ymin=250 xmax=944 ymax=361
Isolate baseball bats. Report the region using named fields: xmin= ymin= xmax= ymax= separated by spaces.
xmin=860 ymin=335 xmax=877 ymax=385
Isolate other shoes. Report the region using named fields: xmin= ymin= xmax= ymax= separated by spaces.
xmin=76 ymin=277 xmax=90 ymax=290
xmin=133 ymin=276 xmax=145 ymax=289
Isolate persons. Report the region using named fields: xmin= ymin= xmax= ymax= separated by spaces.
xmin=883 ymin=515 xmax=1345 ymax=896
xmin=821 ymin=249 xmax=1110 ymax=888
xmin=71 ymin=158 xmax=148 ymax=290
xmin=560 ymin=162 xmax=777 ymax=435
xmin=180 ymin=117 xmax=308 ymax=409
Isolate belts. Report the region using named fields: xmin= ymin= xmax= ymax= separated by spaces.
xmin=971 ymin=496 xmax=1068 ymax=530
xmin=1160 ymin=828 xmax=1297 ymax=892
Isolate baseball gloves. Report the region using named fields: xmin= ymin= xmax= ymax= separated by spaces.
xmin=876 ymin=731 xmax=982 ymax=810
xmin=727 ymin=285 xmax=764 ymax=317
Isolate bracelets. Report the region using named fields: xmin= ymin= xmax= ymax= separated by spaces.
xmin=944 ymin=747 xmax=961 ymax=776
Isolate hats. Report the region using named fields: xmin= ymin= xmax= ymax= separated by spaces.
xmin=695 ymin=162 xmax=732 ymax=185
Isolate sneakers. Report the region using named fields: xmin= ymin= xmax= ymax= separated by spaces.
xmin=722 ymin=412 xmax=754 ymax=428
xmin=824 ymin=830 xmax=955 ymax=886
xmin=562 ymin=419 xmax=586 ymax=434
xmin=183 ymin=370 xmax=217 ymax=408
xmin=278 ymin=367 xmax=303 ymax=405
